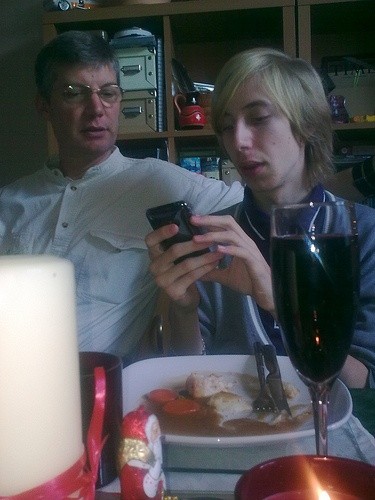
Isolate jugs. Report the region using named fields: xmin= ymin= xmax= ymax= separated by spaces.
xmin=174 ymin=94 xmax=206 ymax=130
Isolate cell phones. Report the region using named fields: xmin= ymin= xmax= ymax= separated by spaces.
xmin=145 ymin=201 xmax=214 ymax=265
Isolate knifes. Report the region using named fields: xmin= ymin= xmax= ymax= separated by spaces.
xmin=263 ymin=344 xmax=291 ymax=415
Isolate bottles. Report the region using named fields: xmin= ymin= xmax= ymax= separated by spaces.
xmin=328 ymin=95 xmax=349 ymax=124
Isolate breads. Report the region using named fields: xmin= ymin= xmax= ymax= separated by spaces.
xmin=186 ymin=373 xmax=299 ymax=416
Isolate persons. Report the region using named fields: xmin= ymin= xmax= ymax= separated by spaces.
xmin=1 ymin=30 xmax=375 ymax=368
xmin=144 ymin=46 xmax=375 ymax=438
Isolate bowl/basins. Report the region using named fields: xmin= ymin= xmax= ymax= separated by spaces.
xmin=116 ymin=354 xmax=354 ymax=472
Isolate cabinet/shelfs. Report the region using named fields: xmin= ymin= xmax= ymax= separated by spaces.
xmin=40 ymin=0 xmax=375 ymax=205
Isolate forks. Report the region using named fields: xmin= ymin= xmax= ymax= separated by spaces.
xmin=253 ymin=341 xmax=277 ymax=412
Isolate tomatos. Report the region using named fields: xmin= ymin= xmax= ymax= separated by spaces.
xmin=162 ymin=399 xmax=200 ymax=414
xmin=149 ymin=389 xmax=177 ymax=402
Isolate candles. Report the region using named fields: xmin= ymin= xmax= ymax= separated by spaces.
xmin=0 ymin=253 xmax=82 ymax=497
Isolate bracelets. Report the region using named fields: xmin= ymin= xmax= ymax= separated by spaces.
xmin=164 ymin=338 xmax=206 ymax=357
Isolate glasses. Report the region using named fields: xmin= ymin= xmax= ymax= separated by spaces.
xmin=54 ymin=81 xmax=126 ymax=105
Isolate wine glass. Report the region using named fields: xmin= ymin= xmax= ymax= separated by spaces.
xmin=268 ymin=200 xmax=360 ymax=459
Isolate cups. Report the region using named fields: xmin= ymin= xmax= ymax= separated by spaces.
xmin=77 ymin=350 xmax=121 ymax=487
